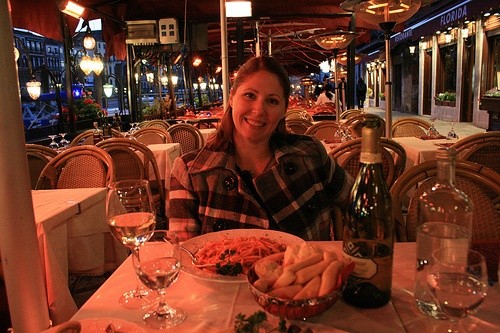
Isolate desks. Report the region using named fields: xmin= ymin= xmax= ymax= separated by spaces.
xmin=393 ymin=137 xmax=468 ymax=211
xmin=69 ymin=241 xmax=500 ymax=333
xmin=200 ymin=128 xmax=216 ymax=148
xmin=134 ymin=142 xmax=183 ymax=217
xmin=31 ymin=187 xmax=110 ymax=323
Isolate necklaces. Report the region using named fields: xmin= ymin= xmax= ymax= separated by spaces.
xmin=239 ymin=160 xmax=266 ymax=178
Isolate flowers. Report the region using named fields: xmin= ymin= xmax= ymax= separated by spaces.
xmin=54 ymin=90 xmax=108 ymax=124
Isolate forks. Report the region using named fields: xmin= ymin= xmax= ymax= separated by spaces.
xmin=164 ymin=236 xmax=216 ymax=268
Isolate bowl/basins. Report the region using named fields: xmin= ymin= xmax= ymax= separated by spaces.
xmin=247 ymin=252 xmax=341 ymax=320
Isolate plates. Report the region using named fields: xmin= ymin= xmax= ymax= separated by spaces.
xmin=173 ymin=229 xmax=306 ymax=283
xmin=40 ymin=317 xmax=144 ymax=333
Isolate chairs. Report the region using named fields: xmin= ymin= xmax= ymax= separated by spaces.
xmin=25 ymin=92 xmax=500 ymax=290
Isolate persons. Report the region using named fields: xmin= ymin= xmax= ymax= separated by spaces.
xmin=169 ymin=55 xmax=355 ymax=242
xmin=355 ymin=78 xmax=366 ymax=108
xmin=315 ymin=79 xmax=335 ymax=98
xmin=338 ymin=77 xmax=346 ymax=102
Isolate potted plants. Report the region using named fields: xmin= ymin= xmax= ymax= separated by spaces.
xmin=434 ymin=92 xmax=456 ymax=107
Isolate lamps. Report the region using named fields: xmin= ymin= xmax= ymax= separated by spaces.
xmin=26 ymin=0 xmax=221 ymax=100
xmin=445 ymin=32 xmax=457 ymax=52
xmin=461 ymin=28 xmax=474 ymax=48
xmin=315 ymin=0 xmax=423 ymax=82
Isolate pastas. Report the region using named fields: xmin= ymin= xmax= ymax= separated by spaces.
xmin=196 ymin=237 xmax=287 ymax=274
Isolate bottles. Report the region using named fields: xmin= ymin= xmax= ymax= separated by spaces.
xmin=115 ymin=112 xmax=121 ymax=127
xmin=341 ymin=120 xmax=393 ymax=309
xmin=102 ymin=117 xmax=111 ymax=136
xmin=414 ymin=150 xmax=473 ymax=320
xmin=110 ymin=116 xmax=121 ymax=132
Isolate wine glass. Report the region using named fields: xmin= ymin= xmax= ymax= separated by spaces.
xmin=176 ymin=120 xmax=188 ymax=124
xmin=446 ymin=119 xmax=457 ymax=141
xmin=343 ymin=126 xmax=353 ymax=142
xmin=427 ymin=247 xmax=488 ymax=333
xmin=132 ymin=230 xmax=187 ymax=330
xmin=426 ymin=117 xmax=440 ymax=142
xmin=105 ymin=179 xmax=156 ymax=310
xmin=130 ymin=122 xmax=141 ymax=134
xmin=334 ymin=122 xmax=345 ymax=142
xmin=48 ymin=135 xmax=59 ymax=149
xmin=60 ymin=133 xmax=69 ymax=150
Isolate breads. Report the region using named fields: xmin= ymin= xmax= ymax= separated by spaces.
xmin=253 ymin=242 xmax=342 ymax=300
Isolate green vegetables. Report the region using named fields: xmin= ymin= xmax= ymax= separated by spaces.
xmin=216 ymin=250 xmax=244 ymax=276
xmin=233 ymin=310 xmax=308 ymax=333
